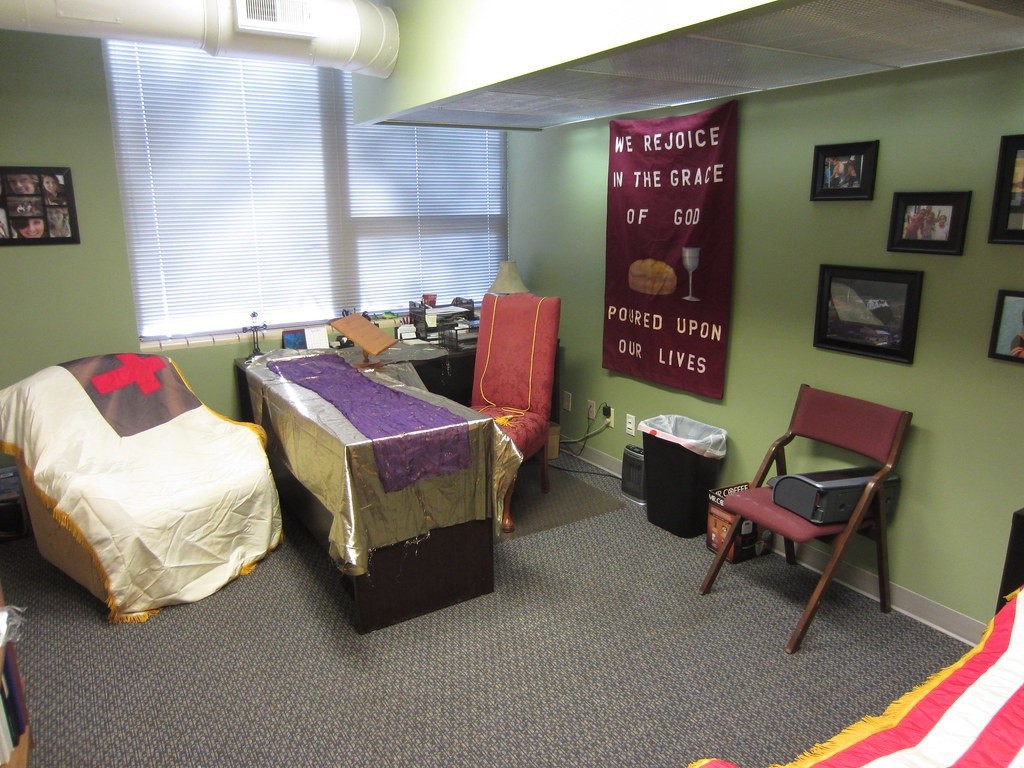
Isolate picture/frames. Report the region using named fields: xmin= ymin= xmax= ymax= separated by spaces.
xmin=986 ymin=289 xmax=1024 ymax=362
xmin=0 ymin=165 xmax=81 ymax=246
xmin=812 ymin=264 xmax=924 ymax=367
xmin=988 ymin=134 xmax=1024 ymax=245
xmin=810 ymin=139 xmax=882 ymax=201
xmin=886 ymin=190 xmax=971 ymax=255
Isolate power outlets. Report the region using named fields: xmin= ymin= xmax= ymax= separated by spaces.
xmin=603 ymin=407 xmax=615 ymax=429
xmin=562 ymin=391 xmax=572 ymax=412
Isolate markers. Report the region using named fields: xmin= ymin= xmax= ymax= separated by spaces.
xmin=400 ymin=320 xmax=403 ymax=325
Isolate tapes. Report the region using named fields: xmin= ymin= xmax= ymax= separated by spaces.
xmin=342 ymin=337 xmax=347 ymax=343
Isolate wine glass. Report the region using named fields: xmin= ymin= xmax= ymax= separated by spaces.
xmin=682 ymin=246 xmax=701 ymax=301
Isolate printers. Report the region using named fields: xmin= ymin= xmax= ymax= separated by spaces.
xmin=766 ymin=465 xmax=902 ymax=525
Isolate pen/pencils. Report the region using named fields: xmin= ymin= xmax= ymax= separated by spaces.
xmin=388 ymin=348 xmax=400 ymax=350
xmin=423 ymin=299 xmax=424 ymax=307
xmin=353 ymin=308 xmax=356 ymax=314
xmin=418 ymin=302 xmax=432 ymax=309
xmin=403 ymin=317 xmax=406 ymax=324
xmin=406 ymin=316 xmax=409 ymax=324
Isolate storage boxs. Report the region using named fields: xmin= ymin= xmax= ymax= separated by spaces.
xmin=706 ymin=482 xmax=773 ymax=563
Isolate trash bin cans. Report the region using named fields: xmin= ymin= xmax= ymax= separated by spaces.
xmin=639 ymin=415 xmax=727 ymax=538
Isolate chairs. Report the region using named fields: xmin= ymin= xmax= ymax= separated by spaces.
xmin=464 ymin=293 xmax=562 ymax=536
xmin=700 ymin=382 xmax=914 ymax=653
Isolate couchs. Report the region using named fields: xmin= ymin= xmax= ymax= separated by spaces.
xmin=0 ymin=351 xmax=284 ymax=622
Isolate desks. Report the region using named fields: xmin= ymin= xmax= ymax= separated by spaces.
xmin=235 ymin=300 xmax=559 ymax=634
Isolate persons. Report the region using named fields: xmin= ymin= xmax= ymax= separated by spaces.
xmin=1010 ymin=311 xmax=1024 ymax=358
xmin=904 ymin=206 xmax=948 ymax=241
xmin=0 ymin=174 xmax=72 ymax=238
xmin=825 ymin=157 xmax=857 ymax=188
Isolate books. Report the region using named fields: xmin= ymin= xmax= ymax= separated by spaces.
xmin=454 ymin=320 xmax=479 ymax=333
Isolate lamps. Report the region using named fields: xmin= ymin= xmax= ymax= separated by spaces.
xmin=486 ymin=260 xmax=530 ymax=293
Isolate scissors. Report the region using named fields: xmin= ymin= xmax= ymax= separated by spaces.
xmin=342 ymin=309 xmax=351 ymax=317
